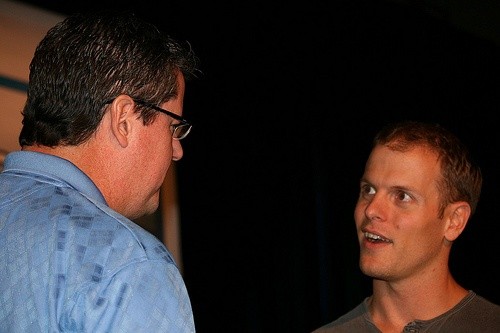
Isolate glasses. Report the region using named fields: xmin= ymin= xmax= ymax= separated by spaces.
xmin=106 ymin=98 xmax=193 ymax=141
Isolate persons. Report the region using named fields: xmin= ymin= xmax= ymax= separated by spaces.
xmin=0 ymin=11 xmax=197 ymax=332
xmin=311 ymin=121 xmax=500 ymax=332
